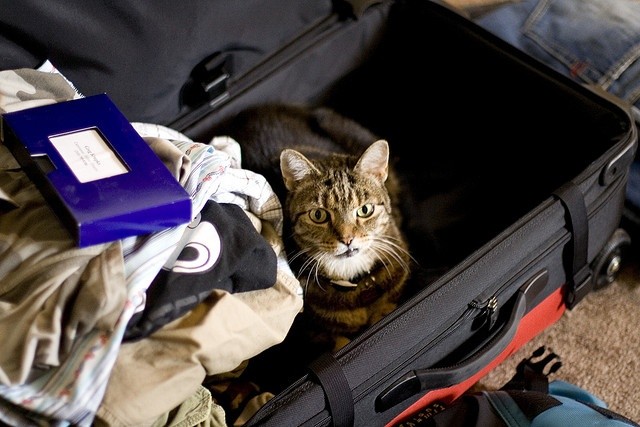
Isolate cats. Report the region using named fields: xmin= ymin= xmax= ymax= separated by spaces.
xmin=218 ymin=100 xmax=418 ymax=354
